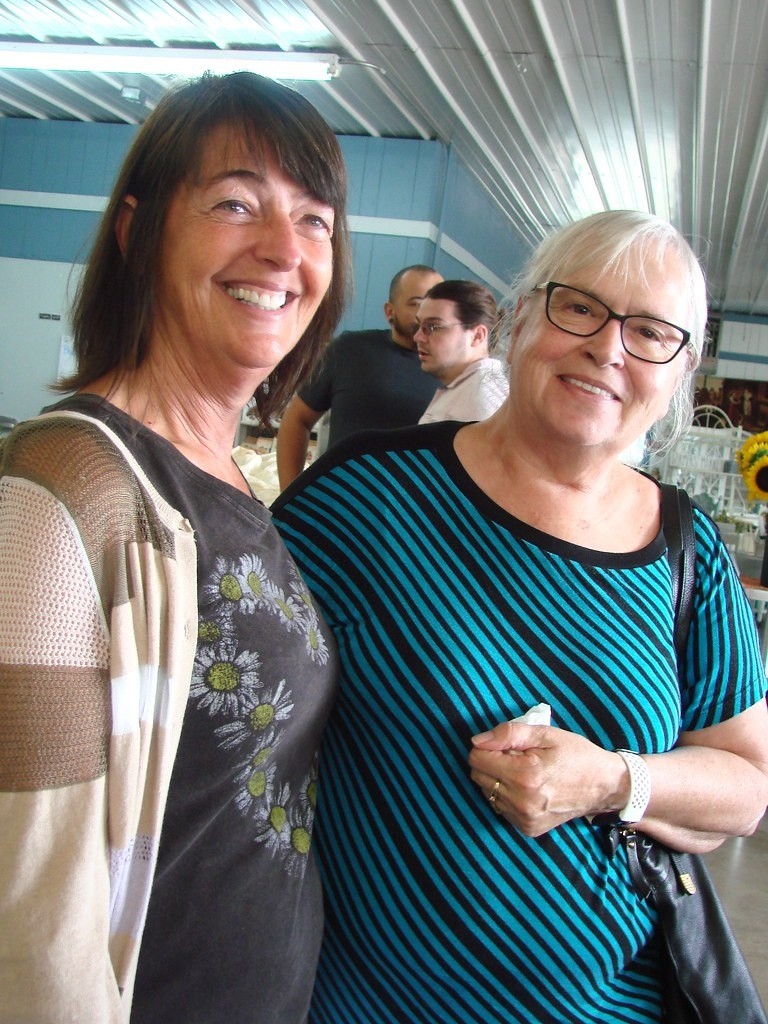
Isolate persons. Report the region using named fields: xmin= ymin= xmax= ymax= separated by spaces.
xmin=409 ymin=280 xmax=509 ymax=424
xmin=267 ymin=208 xmax=768 ymax=1024
xmin=275 ymin=264 xmax=446 ymax=492
xmin=0 ymin=71 xmax=352 ymax=1024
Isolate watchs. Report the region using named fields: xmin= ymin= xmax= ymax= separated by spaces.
xmin=584 ymin=748 xmax=651 ymax=825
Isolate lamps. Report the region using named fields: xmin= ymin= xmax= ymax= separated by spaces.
xmin=0 ymin=39 xmax=342 ymax=81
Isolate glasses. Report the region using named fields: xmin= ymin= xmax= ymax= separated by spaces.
xmin=411 ymin=321 xmax=480 ymax=335
xmin=530 ymin=281 xmax=698 ymax=365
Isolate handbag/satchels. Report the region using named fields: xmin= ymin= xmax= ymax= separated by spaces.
xmin=621 ymin=483 xmax=767 ymax=1024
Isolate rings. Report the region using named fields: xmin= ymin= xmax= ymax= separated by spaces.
xmin=489 ymin=782 xmax=500 ymax=801
